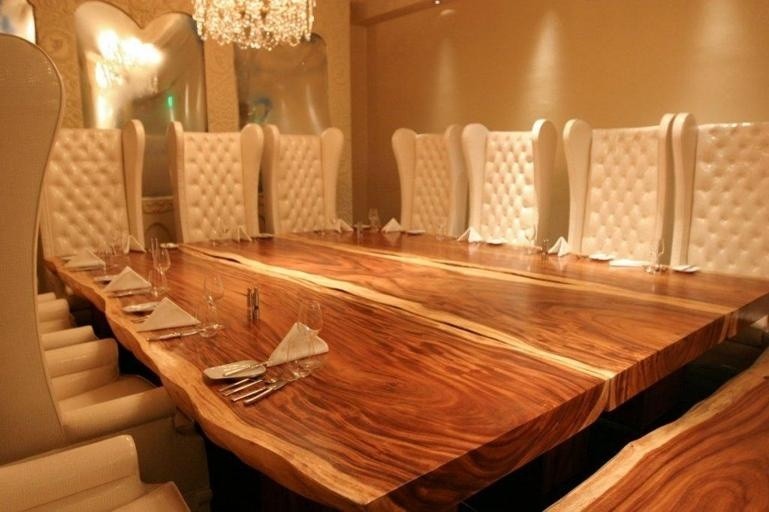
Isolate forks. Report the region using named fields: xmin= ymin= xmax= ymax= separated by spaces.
xmin=230 ymin=377 xmax=286 ymax=402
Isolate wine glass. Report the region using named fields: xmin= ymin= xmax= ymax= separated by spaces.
xmin=297 ymin=300 xmax=324 ymax=370
xmin=203 ymin=273 xmax=222 ymax=329
xmin=368 ymin=208 xmax=378 ymax=233
xmin=649 ymin=238 xmax=665 ymax=272
xmin=153 ymin=248 xmax=171 ymax=291
xmin=524 ymin=223 xmax=535 ymax=255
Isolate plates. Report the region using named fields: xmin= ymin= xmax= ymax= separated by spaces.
xmin=671 ymin=264 xmax=699 ymax=272
xmin=122 ymin=301 xmax=160 ymax=313
xmin=590 ymin=254 xmax=614 ymax=262
xmin=93 ymin=275 xmax=116 ymax=282
xmin=409 ymin=229 xmax=426 ymax=234
xmin=204 ymin=360 xmax=267 ymax=380
xmin=487 ymin=238 xmax=507 ymax=245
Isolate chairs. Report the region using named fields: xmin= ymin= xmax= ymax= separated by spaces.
xmin=558 ymin=113 xmax=678 ymax=263
xmin=461 ymin=119 xmax=557 ymax=241
xmin=387 ymin=121 xmax=467 ymax=228
xmin=166 ymin=121 xmax=266 ymax=239
xmin=0 ymin=36 xmax=217 ymax=512
xmin=264 ymin=125 xmax=347 ymax=230
xmin=667 ymin=111 xmax=765 ymax=378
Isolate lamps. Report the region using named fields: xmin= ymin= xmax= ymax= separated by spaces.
xmin=192 ymin=0 xmax=316 ymax=52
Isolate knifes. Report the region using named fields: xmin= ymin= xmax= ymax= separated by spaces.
xmin=147 ymin=330 xmax=202 ymax=341
xmin=243 ymin=380 xmax=291 ymax=407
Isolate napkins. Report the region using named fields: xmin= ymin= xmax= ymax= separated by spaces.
xmin=330 ymin=220 xmax=354 ymax=234
xmin=547 ymin=236 xmax=572 ymax=257
xmin=381 ymin=217 xmax=402 ymax=232
xmin=266 ymin=324 xmax=327 ymax=369
xmin=227 ymin=225 xmax=253 ymax=243
xmin=457 ymin=229 xmax=481 ymax=243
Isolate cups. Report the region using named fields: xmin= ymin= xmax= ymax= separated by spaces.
xmin=195 ymin=300 xmax=220 ymax=338
